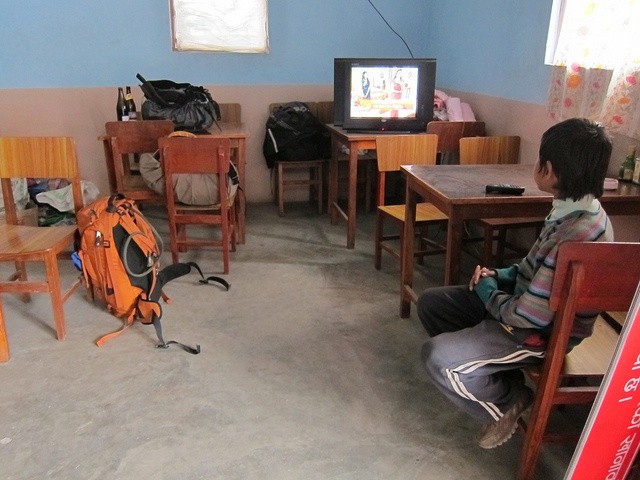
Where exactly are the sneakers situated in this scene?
[37,205,62,223]
[478,385,534,449]
[497,370,525,385]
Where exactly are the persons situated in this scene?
[417,117,614,450]
[390,69,404,118]
[360,71,372,100]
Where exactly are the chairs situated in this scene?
[512,241,640,480]
[373,134,449,269]
[217,103,241,165]
[426,121,485,232]
[158,137,237,274]
[0,136,96,339]
[318,101,372,212]
[269,101,324,217]
[460,136,547,268]
[105,121,188,253]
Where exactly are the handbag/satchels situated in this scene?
[142,81,222,133]
[138,131,247,225]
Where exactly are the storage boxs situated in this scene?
[0,199,40,227]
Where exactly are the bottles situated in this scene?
[116,88,129,126]
[617,147,635,183]
[125,88,137,122]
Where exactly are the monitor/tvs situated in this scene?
[334,58,436,134]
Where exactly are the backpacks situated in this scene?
[262,102,330,195]
[70,192,230,355]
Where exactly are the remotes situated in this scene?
[486,185,525,195]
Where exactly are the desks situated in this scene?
[400,164,640,319]
[97,122,250,245]
[324,123,427,250]
